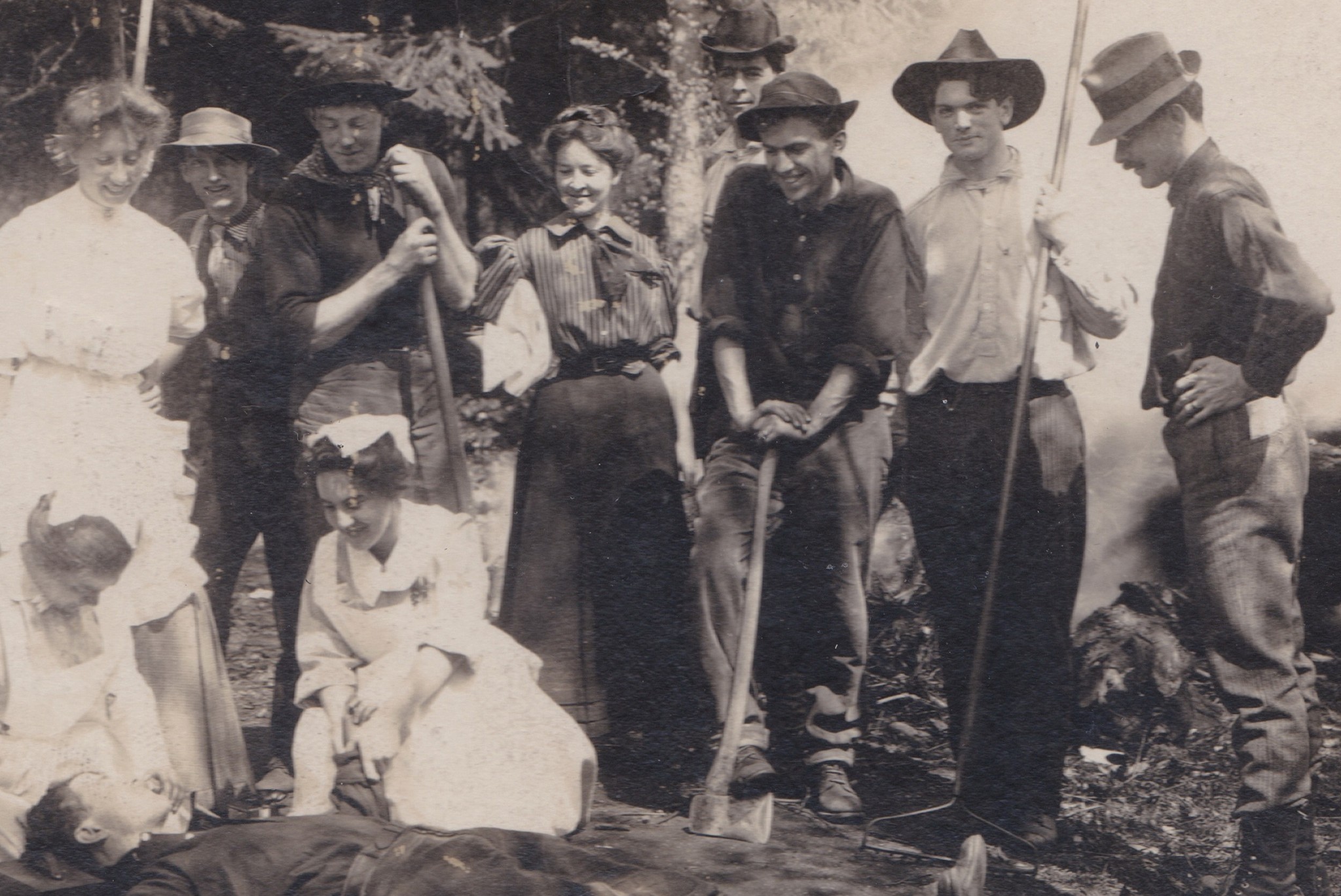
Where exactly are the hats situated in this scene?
[698,1,797,57]
[893,29,1046,131]
[276,46,419,108]
[157,107,280,160]
[736,72,860,142]
[1079,31,1202,147]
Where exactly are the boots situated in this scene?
[1225,813,1306,896]
[1297,806,1332,896]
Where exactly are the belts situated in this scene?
[342,819,410,896]
[560,352,650,378]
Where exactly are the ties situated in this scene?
[208,224,226,290]
[579,222,664,307]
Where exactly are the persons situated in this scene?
[475,103,718,738]
[900,28,1138,853]
[687,1,787,465]
[164,106,326,777]
[1104,32,1333,894]
[24,771,988,895]
[701,71,907,820]
[290,412,599,836]
[1,76,257,831]
[260,62,478,558]
[0,487,178,866]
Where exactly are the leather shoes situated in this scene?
[733,746,777,784]
[1015,814,1058,849]
[812,761,867,817]
